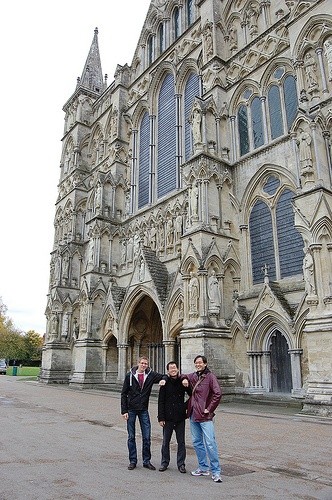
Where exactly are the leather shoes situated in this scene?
[128,463,136,470]
[178,464,186,473]
[143,463,156,470]
[159,462,169,471]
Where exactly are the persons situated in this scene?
[158,361,192,472]
[62,257,69,275]
[189,272,199,312]
[180,355,222,482]
[96,184,101,206]
[51,312,69,333]
[295,128,313,168]
[324,40,332,78]
[190,183,198,216]
[303,248,316,296]
[205,29,212,55]
[193,111,201,143]
[207,271,221,309]
[302,53,317,88]
[143,212,182,249]
[121,358,167,470]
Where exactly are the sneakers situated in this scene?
[191,468,210,476]
[211,474,223,482]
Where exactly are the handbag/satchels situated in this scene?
[184,398,189,409]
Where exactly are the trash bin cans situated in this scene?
[13,366,17,376]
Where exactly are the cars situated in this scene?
[0,360,7,375]
